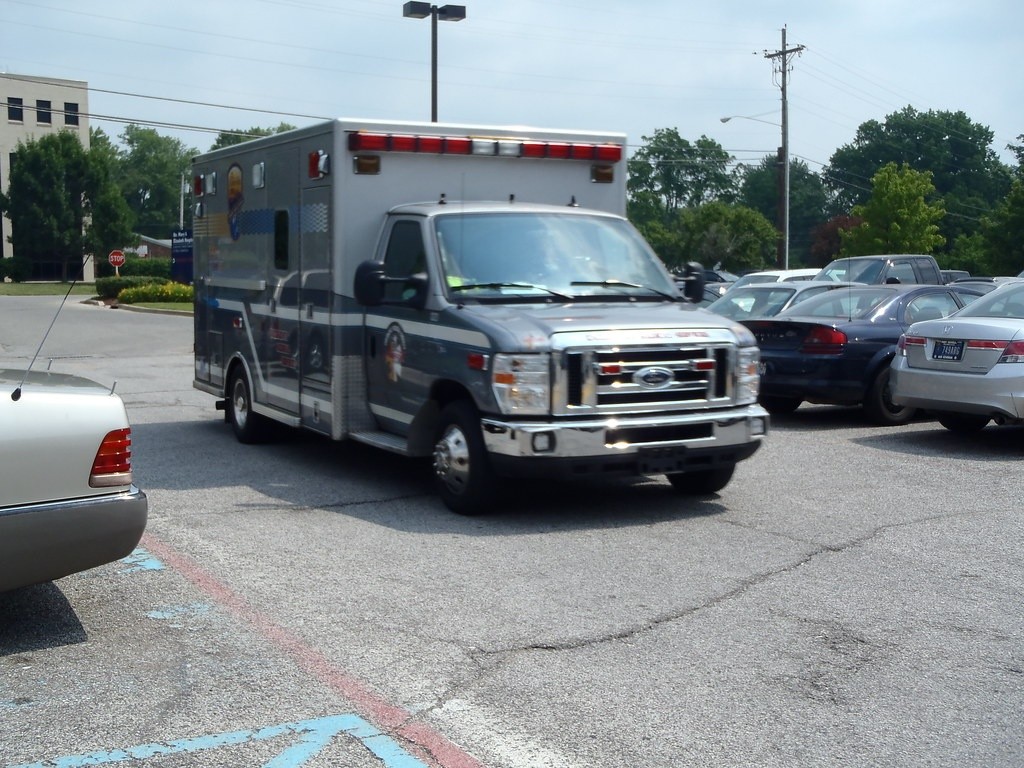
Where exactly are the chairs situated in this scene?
[913,306,943,322]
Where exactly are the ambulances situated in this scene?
[190,117,769,514]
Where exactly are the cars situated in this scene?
[671,254,1024,435]
[0,368,147,593]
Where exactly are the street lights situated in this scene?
[403,1,466,121]
[722,115,789,268]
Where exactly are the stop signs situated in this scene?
[109,250,125,268]
